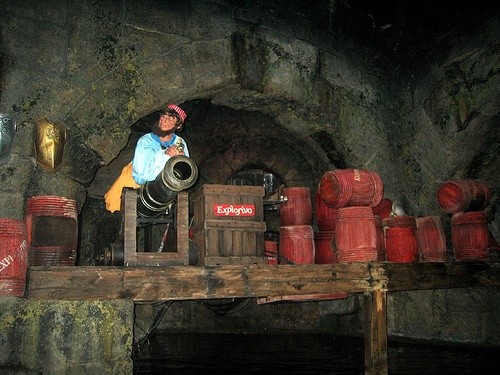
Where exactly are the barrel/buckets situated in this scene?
[318,168,383,208]
[314,230,336,264]
[451,211,491,261]
[279,187,312,226]
[24,195,78,266]
[279,223,315,266]
[373,198,392,219]
[414,216,447,262]
[437,180,491,214]
[383,217,418,263]
[263,230,279,265]
[0,219,29,297]
[373,215,385,261]
[335,206,378,263]
[315,194,337,232]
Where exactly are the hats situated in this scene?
[168,104,187,123]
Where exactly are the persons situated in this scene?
[131,104,191,253]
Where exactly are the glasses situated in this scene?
[161,114,174,122]
